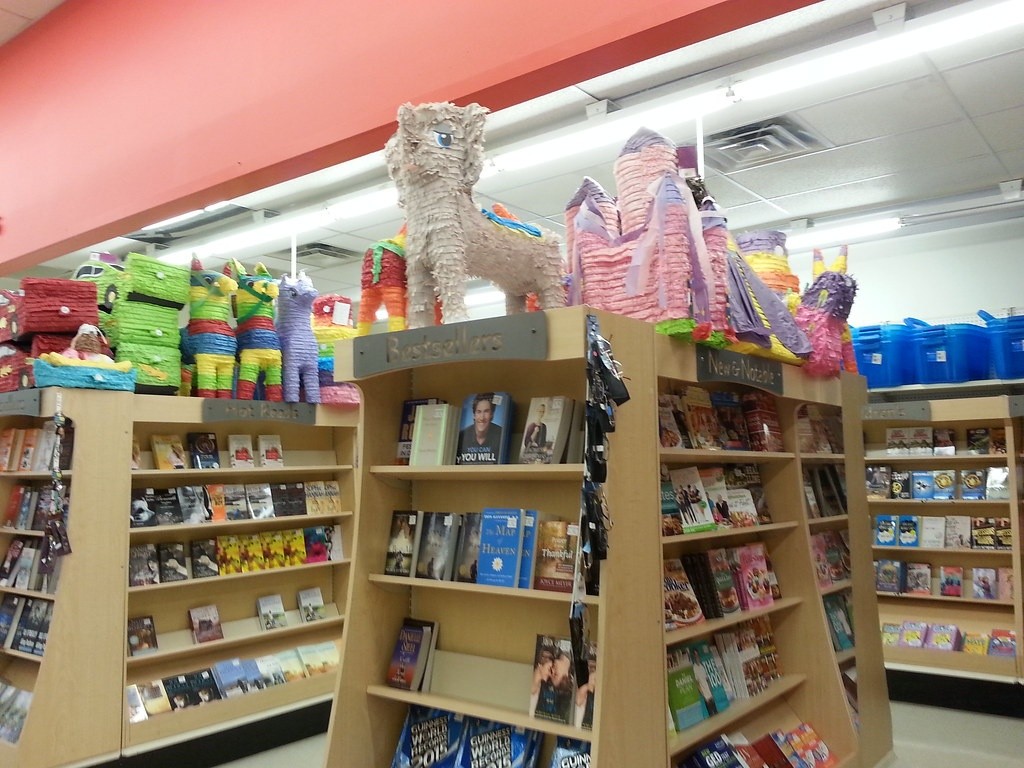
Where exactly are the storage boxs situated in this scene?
[840,309,1023,391]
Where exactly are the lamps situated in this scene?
[784,217,908,251]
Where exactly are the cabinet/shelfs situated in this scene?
[652,331,895,768]
[2,391,133,767]
[121,394,363,759]
[857,396,1023,683]
[318,303,666,767]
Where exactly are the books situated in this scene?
[863,419,1017,658]
[382,391,600,768]
[0,422,76,745]
[658,375,862,768]
[128,432,345,724]
